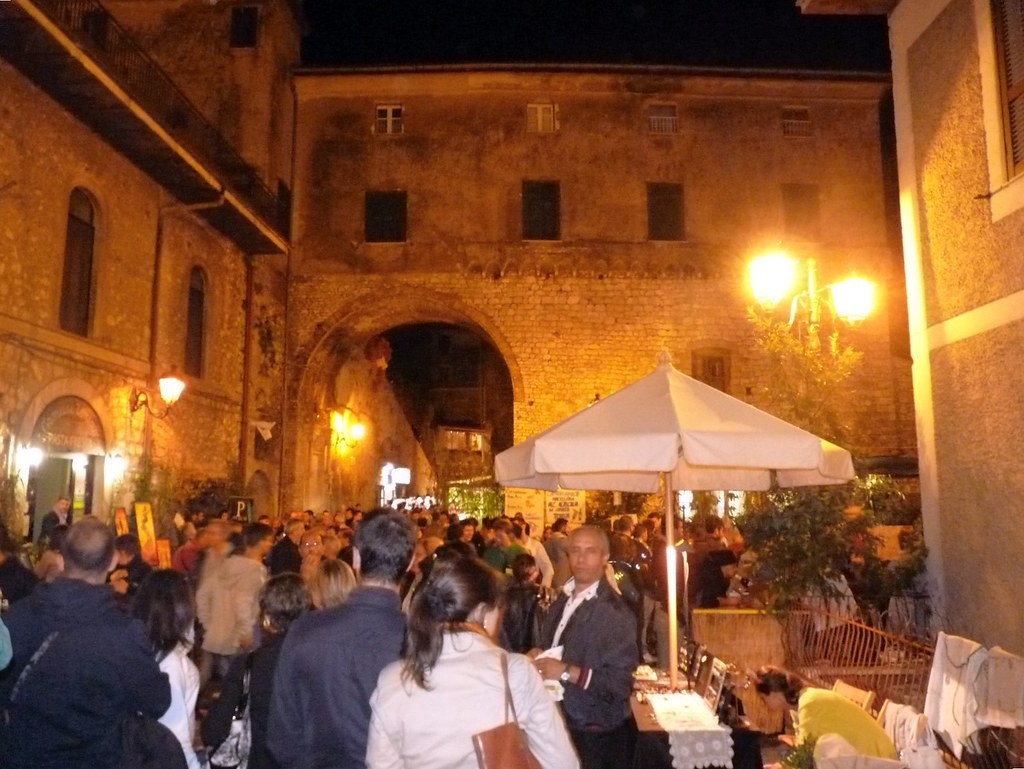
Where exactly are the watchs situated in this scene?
[560,663,572,686]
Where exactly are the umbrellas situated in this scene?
[494,351,857,691]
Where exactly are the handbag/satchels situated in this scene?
[532,585,555,646]
[207,649,252,769]
[472,654,543,769]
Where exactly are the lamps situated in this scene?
[130,364,187,420]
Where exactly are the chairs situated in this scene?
[703,656,727,711]
[876,699,924,748]
[777,679,876,751]
[686,642,706,686]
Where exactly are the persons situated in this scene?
[1,494,747,769]
[756,664,900,769]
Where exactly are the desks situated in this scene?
[632,667,687,693]
[630,690,735,769]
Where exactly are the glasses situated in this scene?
[280,532,287,538]
[301,541,322,548]
